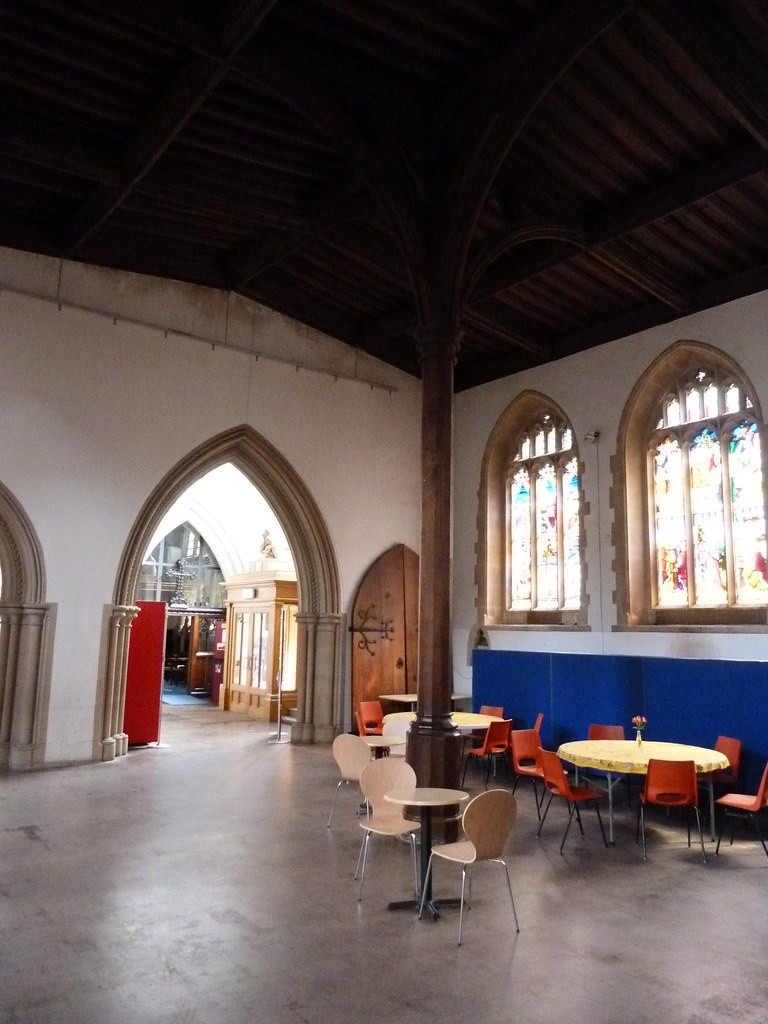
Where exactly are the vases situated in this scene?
[636,731,642,749]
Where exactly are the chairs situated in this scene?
[355,701,389,757]
[536,747,608,854]
[327,734,371,827]
[354,758,422,911]
[713,737,742,784]
[636,759,707,864]
[511,729,568,821]
[714,763,768,857]
[586,724,631,811]
[418,789,520,946]
[460,706,503,769]
[462,719,512,791]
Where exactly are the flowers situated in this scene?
[632,716,648,746]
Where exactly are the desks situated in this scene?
[557,741,730,846]
[360,736,406,760]
[378,694,472,712]
[384,788,469,922]
[382,712,504,781]
